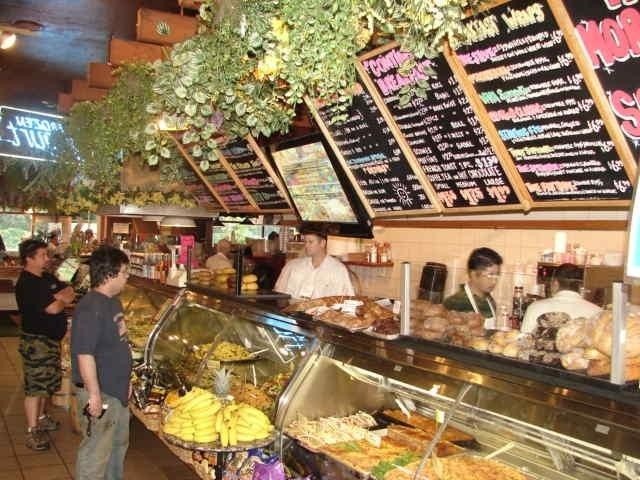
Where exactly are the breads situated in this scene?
[292,297,640,389]
[190,267,259,291]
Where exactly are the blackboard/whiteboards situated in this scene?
[156,0,639,217]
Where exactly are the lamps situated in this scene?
[143,216,197,228]
[0,27,16,50]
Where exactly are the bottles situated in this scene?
[363,245,392,265]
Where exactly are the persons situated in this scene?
[273,232,356,305]
[205,239,235,270]
[442,246,503,330]
[85,229,97,247]
[520,262,604,335]
[47,235,59,248]
[69,245,136,480]
[14,238,75,451]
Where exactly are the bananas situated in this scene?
[152,386,273,449]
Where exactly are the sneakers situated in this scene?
[24,415,60,450]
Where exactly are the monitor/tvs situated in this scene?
[264,132,373,239]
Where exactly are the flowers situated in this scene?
[144,0,488,171]
[52,188,197,214]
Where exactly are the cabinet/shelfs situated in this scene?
[538,263,624,295]
